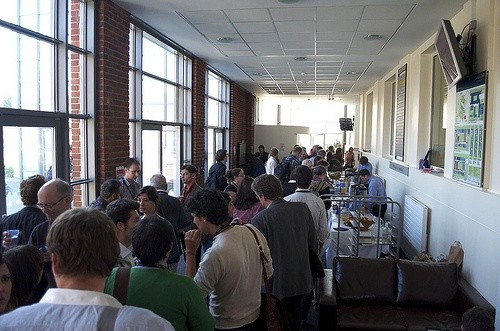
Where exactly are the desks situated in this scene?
[326,196,381,269]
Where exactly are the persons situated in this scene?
[347,158,374,175]
[249,145,374,177]
[178,189,276,331]
[0,254,14,314]
[0,245,44,315]
[264,146,281,175]
[359,168,388,218]
[0,177,74,294]
[283,166,327,257]
[89,180,122,208]
[0,174,47,249]
[135,184,175,272]
[250,152,266,181]
[216,191,235,223]
[118,156,146,201]
[345,146,358,173]
[232,177,264,226]
[176,162,204,274]
[104,196,143,265]
[206,148,229,191]
[151,173,188,272]
[251,174,319,331]
[224,167,248,201]
[0,209,176,331]
[309,166,331,209]
[282,144,303,177]
[108,213,210,331]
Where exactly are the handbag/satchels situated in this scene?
[267,309,283,331]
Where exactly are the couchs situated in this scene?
[318,256,496,331]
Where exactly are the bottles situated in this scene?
[382,223,392,244]
[360,198,366,216]
[333,179,345,194]
[371,217,382,241]
[332,198,338,215]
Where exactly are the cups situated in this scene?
[7,230,19,248]
[341,187,346,193]
[342,208,350,222]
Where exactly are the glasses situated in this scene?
[36,196,68,208]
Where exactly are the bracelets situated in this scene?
[183,249,197,255]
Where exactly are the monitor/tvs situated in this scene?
[435,19,467,89]
